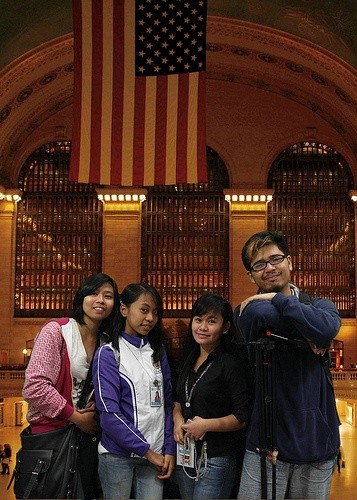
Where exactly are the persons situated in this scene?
[172,293,252,500]
[22,273,120,500]
[0,444,12,475]
[92,282,175,500]
[336,447,343,474]
[233,231,341,500]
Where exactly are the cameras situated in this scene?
[184,419,206,440]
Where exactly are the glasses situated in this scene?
[240,254,288,272]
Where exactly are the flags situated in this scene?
[68,0,208,184]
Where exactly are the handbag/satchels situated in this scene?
[8,424,85,500]
